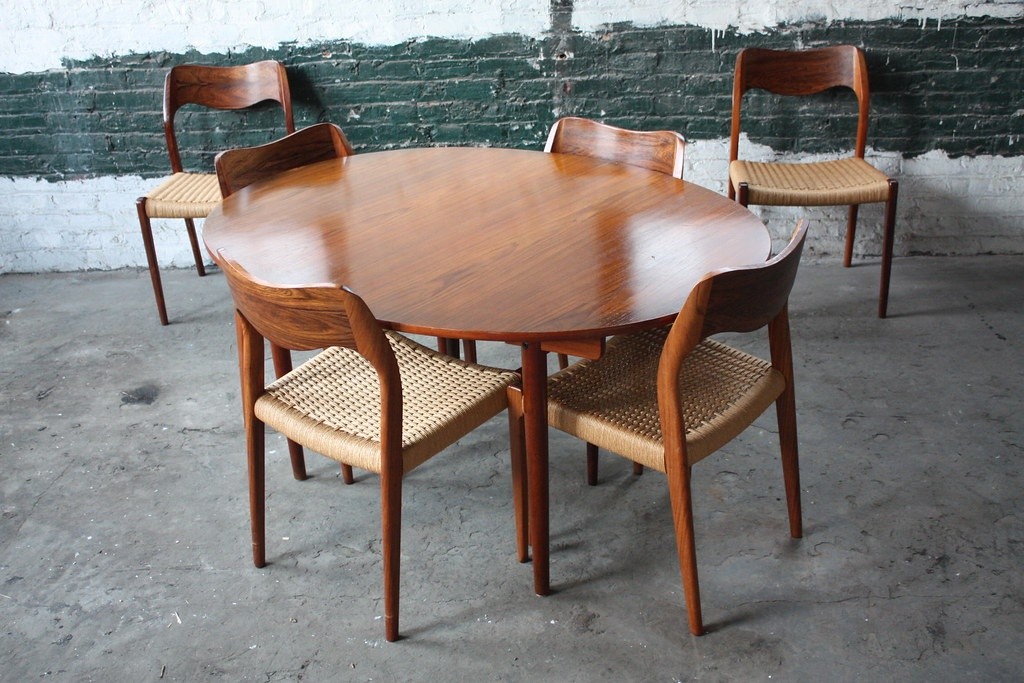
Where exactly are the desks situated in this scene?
[204,145,771,599]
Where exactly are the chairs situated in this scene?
[505,224,808,635]
[216,248,526,642]
[213,120,445,483]
[135,59,298,325]
[462,116,687,487]
[729,46,898,318]
